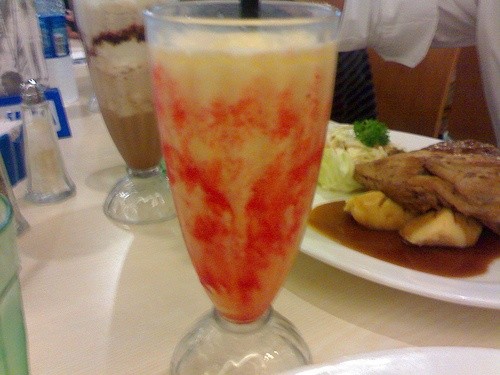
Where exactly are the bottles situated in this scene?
[18,81,75,204]
[34,0,79,109]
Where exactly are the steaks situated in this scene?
[353,139,500,236]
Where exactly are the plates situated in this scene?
[293,346,500,375]
[299,124,500,314]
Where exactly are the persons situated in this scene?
[268,0,499,144]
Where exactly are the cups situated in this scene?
[0,192,29,375]
[139,0,312,375]
[72,0,198,227]
